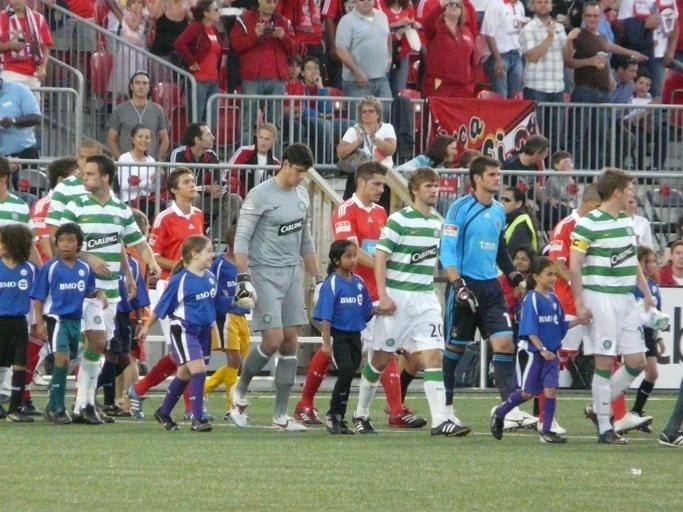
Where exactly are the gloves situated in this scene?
[508,270,528,302]
[312,274,324,305]
[232,272,258,311]
[450,276,480,314]
[638,297,669,331]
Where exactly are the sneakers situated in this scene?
[1,382,683,449]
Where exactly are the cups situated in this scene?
[595,51,611,71]
[263,20,274,34]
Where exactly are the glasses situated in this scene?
[501,197,515,203]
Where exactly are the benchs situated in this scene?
[138,332,325,373]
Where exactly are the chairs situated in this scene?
[660,0,683,132]
[524,184,682,247]
[51,1,572,154]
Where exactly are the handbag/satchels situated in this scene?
[337,126,376,174]
